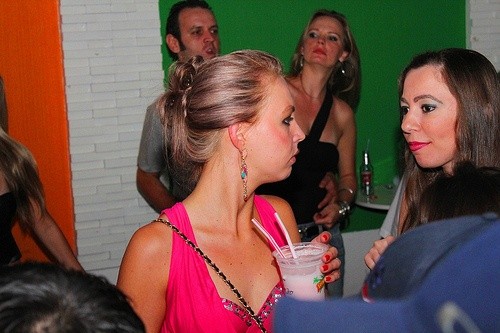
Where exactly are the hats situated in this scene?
[274,207,500,333]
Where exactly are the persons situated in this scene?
[0,0,500,333]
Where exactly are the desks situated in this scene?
[353,183,401,210]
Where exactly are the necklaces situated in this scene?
[300,73,320,98]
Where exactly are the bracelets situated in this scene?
[338,187,355,198]
[336,200,350,217]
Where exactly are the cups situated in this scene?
[273,243,327,302]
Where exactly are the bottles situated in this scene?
[360,152,374,199]
